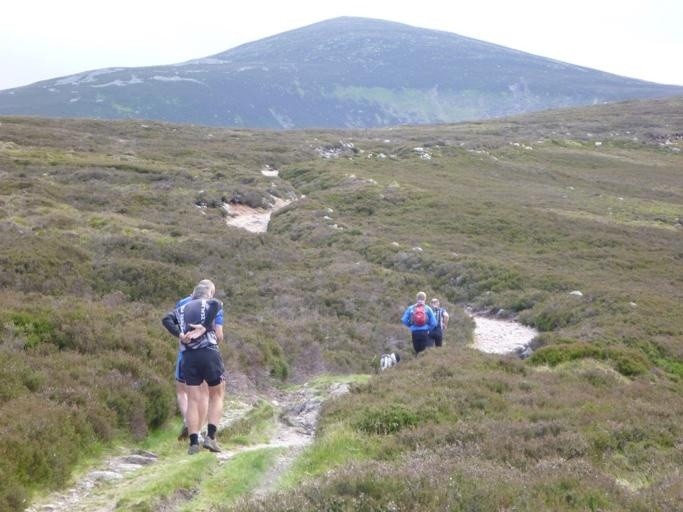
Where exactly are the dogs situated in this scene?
[379,352,400,371]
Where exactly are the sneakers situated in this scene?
[203,437,220,452]
[178,428,188,441]
[188,445,199,454]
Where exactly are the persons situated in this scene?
[174,278,216,442]
[400,291,436,355]
[428,298,449,347]
[161,284,227,454]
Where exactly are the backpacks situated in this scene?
[411,301,428,325]
